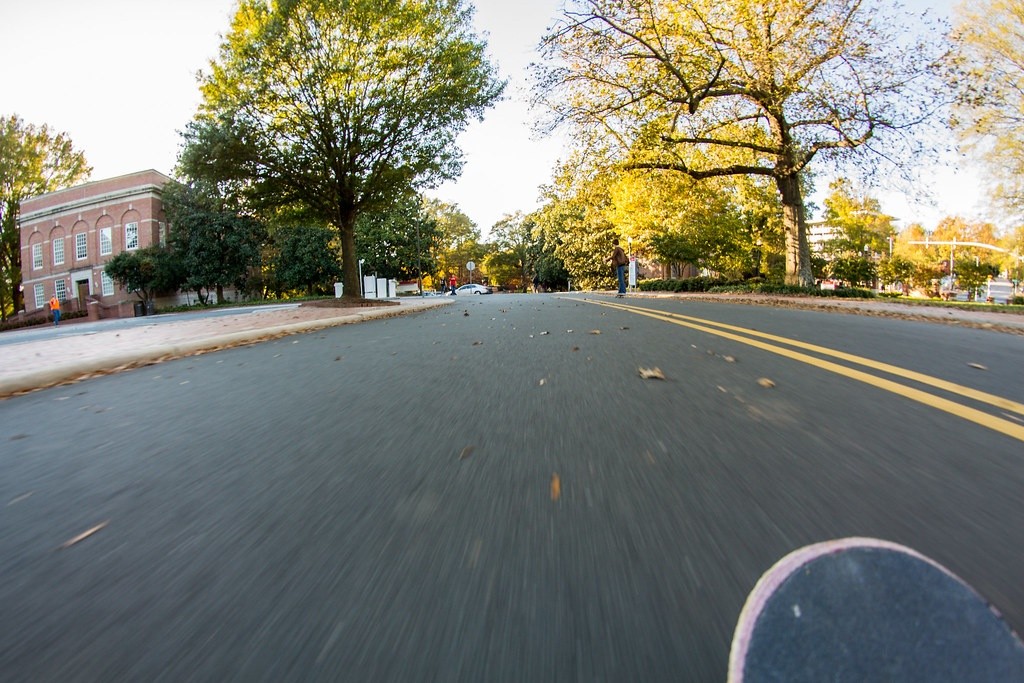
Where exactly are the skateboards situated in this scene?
[615,293,626,298]
[725,536,1024,683]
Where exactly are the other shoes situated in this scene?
[617,293,626,295]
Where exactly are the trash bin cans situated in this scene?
[134,301,146,317]
[145,302,154,316]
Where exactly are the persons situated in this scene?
[50,294,61,325]
[533,274,540,293]
[450,274,457,295]
[605,239,628,294]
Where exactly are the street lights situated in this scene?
[757,240,763,276]
[627,236,632,262]
[359,259,365,297]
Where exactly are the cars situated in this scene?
[446,283,493,294]
